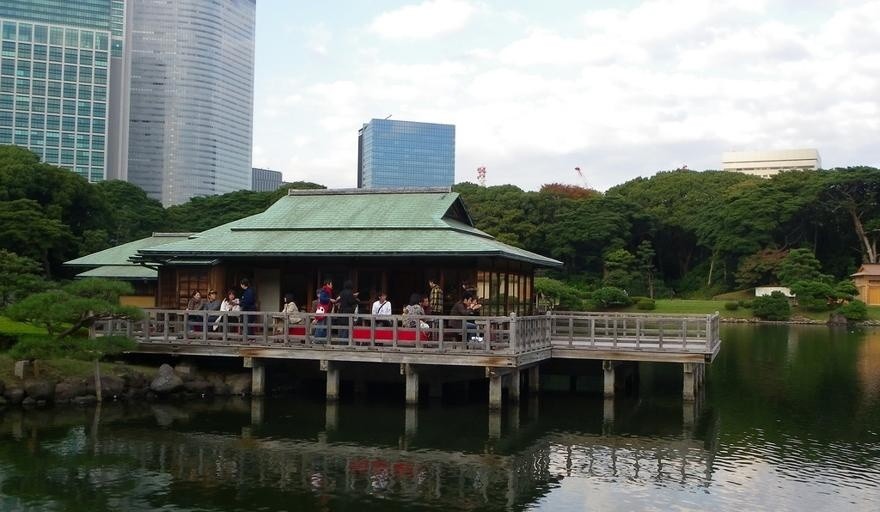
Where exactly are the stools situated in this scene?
[288,326,306,343]
[443,326,461,351]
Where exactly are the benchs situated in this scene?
[351,325,428,350]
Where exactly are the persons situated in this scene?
[214,457,427,497]
[185,277,484,347]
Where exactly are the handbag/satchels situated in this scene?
[212,314,225,331]
[315,303,331,321]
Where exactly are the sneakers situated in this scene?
[248,339,256,343]
[312,339,327,344]
